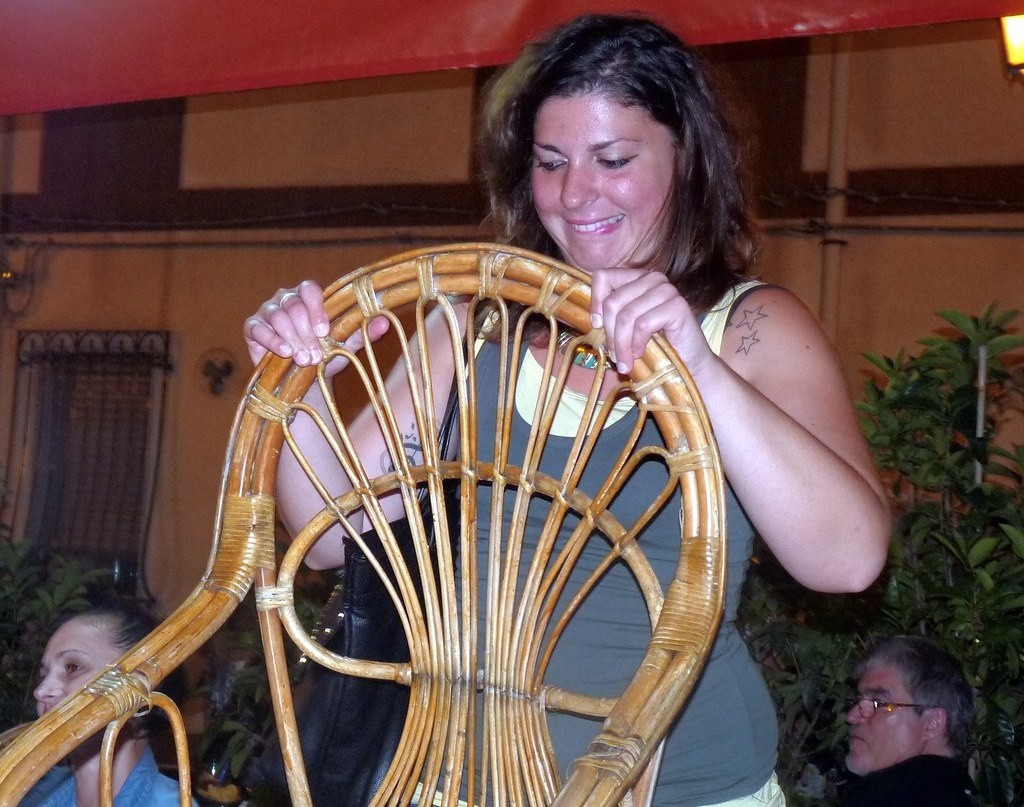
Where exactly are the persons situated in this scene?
[240,9,893,807]
[828,634,986,806]
[18,599,196,806]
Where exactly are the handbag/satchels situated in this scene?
[233,491,463,807]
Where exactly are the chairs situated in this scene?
[0,241,729,807]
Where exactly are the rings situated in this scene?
[262,302,279,317]
[244,317,261,339]
[277,288,299,312]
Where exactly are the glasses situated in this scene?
[844,698,923,719]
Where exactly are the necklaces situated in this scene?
[554,326,617,370]
